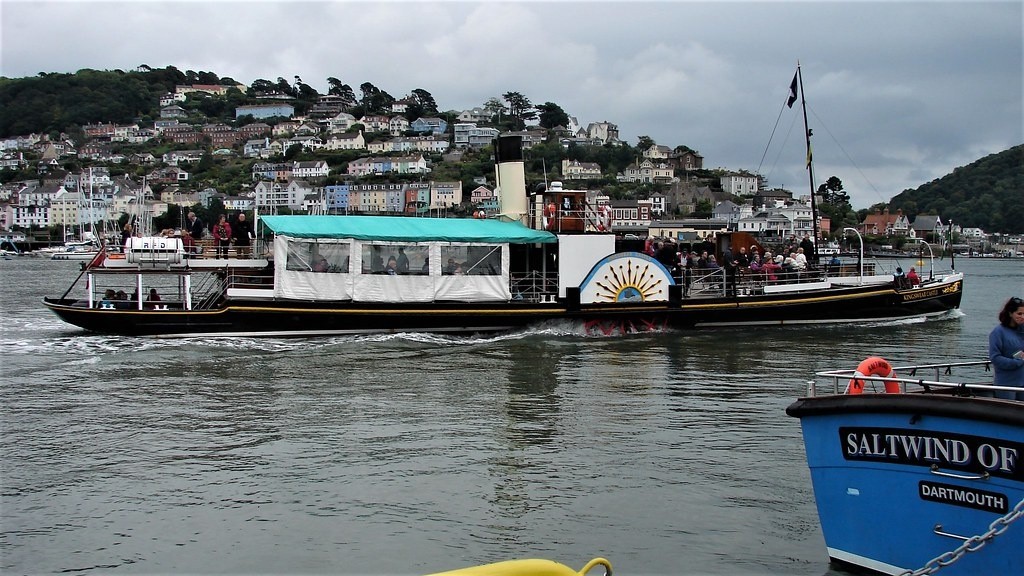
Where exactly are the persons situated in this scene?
[907,267,920,285]
[213,214,231,259]
[643,234,814,297]
[120,224,132,252]
[828,252,840,277]
[422,257,429,275]
[187,212,203,239]
[561,199,569,216]
[99,288,163,310]
[160,228,196,259]
[448,258,480,275]
[231,213,256,259]
[372,246,409,275]
[310,254,328,272]
[989,297,1024,401]
[894,267,905,289]
[341,255,349,273]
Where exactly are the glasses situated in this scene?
[1013,299,1024,304]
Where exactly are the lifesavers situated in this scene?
[596,205,612,231]
[849,358,900,394]
[542,202,556,231]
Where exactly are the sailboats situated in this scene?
[1,165,268,279]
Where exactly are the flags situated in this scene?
[805,139,813,169]
[787,72,797,109]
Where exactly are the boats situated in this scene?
[39,54,966,338]
[785,359,1024,576]
